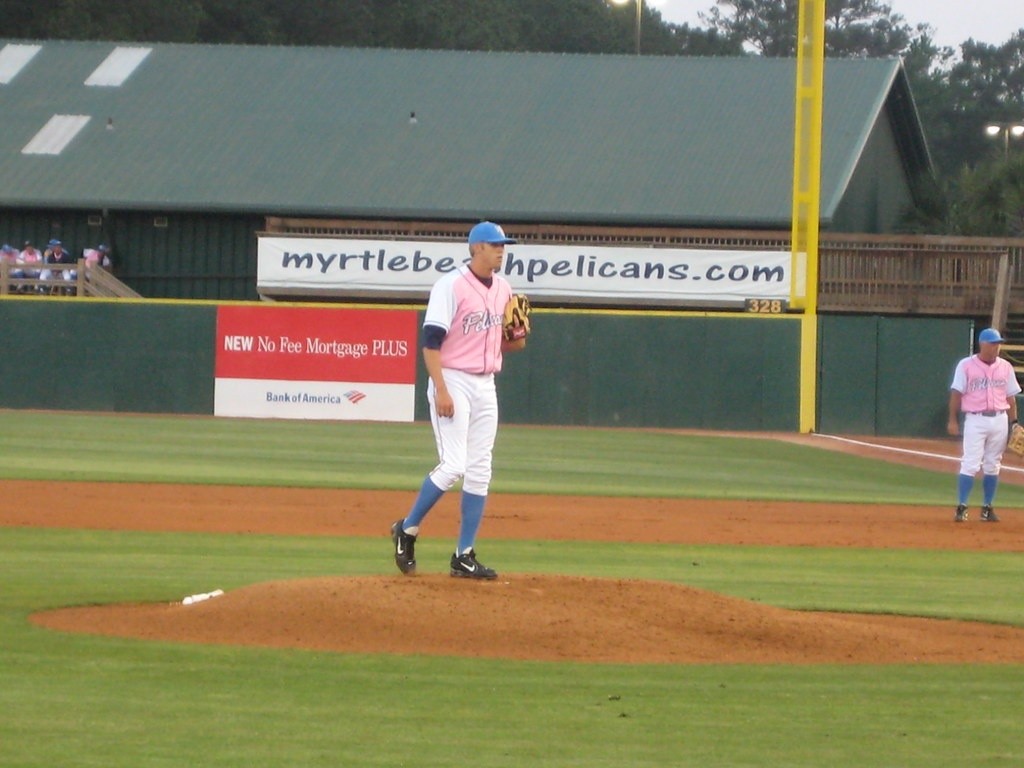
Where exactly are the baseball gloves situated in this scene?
[1007,423,1024,456]
[503,294,531,340]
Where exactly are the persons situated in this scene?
[0,240,110,295]
[947,329,1024,522]
[390,223,531,579]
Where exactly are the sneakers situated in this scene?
[450,548,498,580]
[979,505,1000,522]
[954,504,969,522]
[391,518,419,574]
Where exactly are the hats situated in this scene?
[2,244,12,254]
[54,241,62,247]
[47,239,57,248]
[468,221,517,244]
[99,245,107,252]
[25,241,32,247]
[979,328,1005,343]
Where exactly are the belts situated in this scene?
[972,411,1004,417]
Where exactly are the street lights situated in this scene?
[984,120,1024,162]
